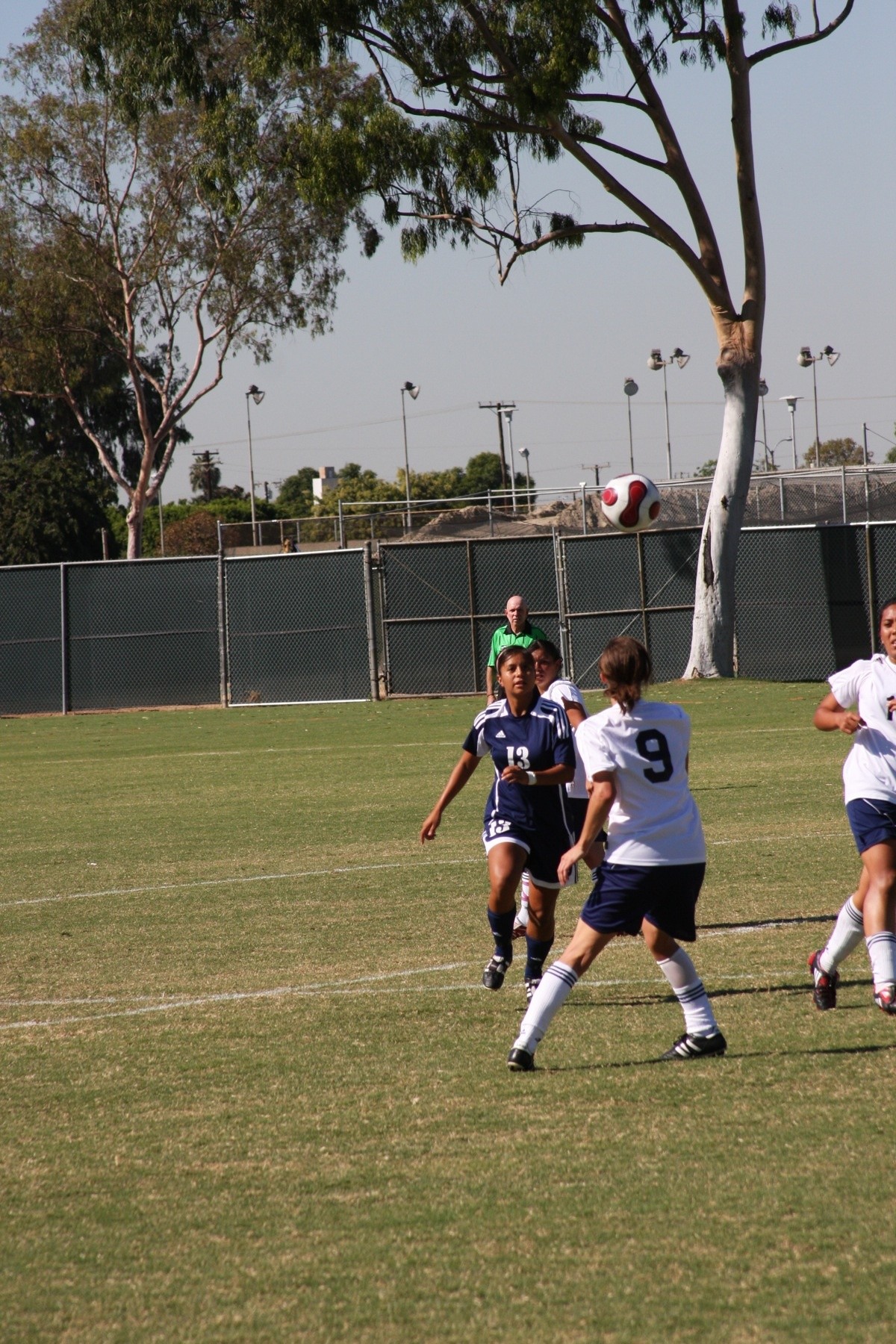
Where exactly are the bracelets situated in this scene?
[526,771,536,785]
[486,693,493,697]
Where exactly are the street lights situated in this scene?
[755,437,793,472]
[758,377,769,472]
[797,345,841,466]
[245,384,265,547]
[579,482,588,535]
[401,381,421,532]
[518,447,532,514]
[497,406,519,515]
[623,377,639,474]
[779,395,804,469]
[647,347,691,478]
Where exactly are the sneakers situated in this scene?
[873,984,896,1016]
[660,1025,727,1060]
[524,976,543,1003]
[512,916,527,940]
[483,954,513,991]
[507,1047,536,1072]
[806,950,840,1012]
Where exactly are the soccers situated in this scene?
[599,473,661,534]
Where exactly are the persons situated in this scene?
[510,644,606,942]
[808,597,896,1012]
[420,646,576,1007]
[507,638,728,1072]
[486,596,547,706]
[279,537,300,554]
